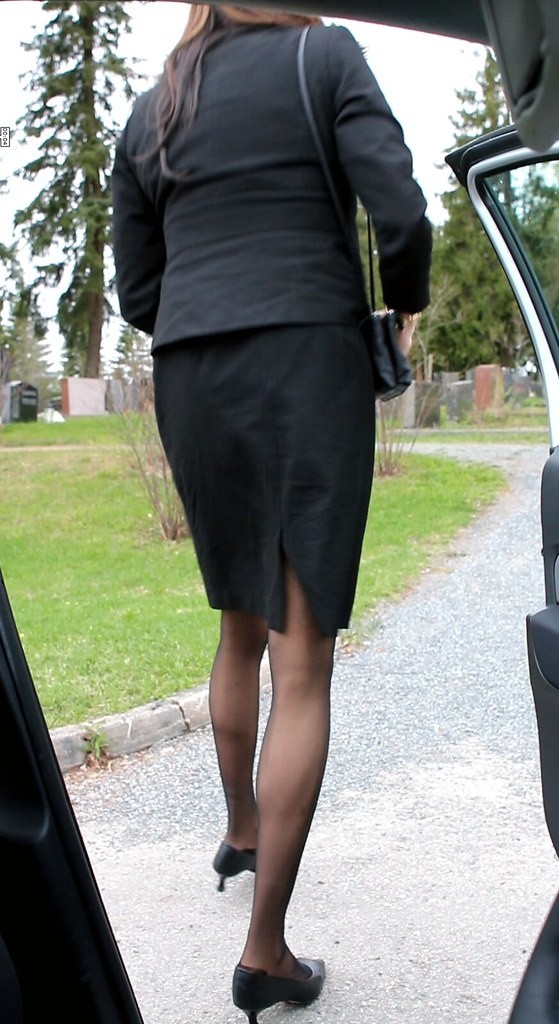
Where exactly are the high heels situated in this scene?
[211,842,256,891]
[231,955,327,1024]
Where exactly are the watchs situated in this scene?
[393,312,424,330]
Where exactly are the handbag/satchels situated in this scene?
[370,307,411,396]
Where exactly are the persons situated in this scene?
[114,3,434,1024]
